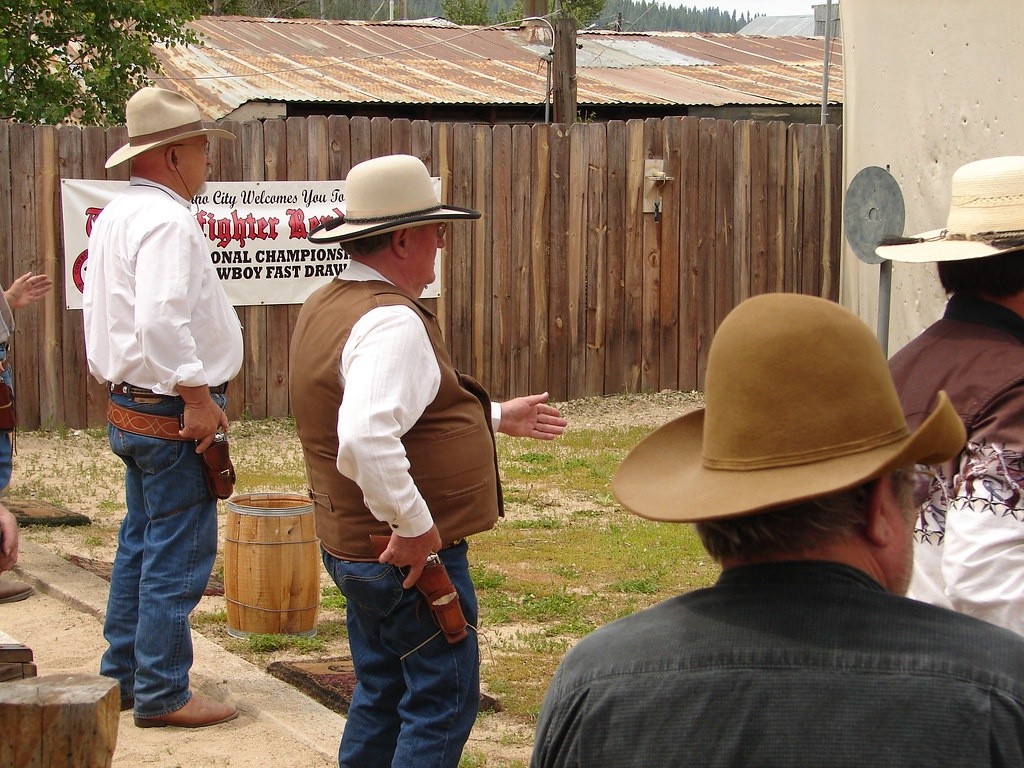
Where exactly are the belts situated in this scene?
[109,382,225,398]
[106,400,195,441]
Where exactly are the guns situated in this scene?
[179,412,226,447]
[369,534,443,579]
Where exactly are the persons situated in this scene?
[523,292,1024,768]
[83,88,243,726]
[888,157,1024,638]
[0,271,53,604]
[289,154,567,768]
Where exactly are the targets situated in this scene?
[843,165,905,265]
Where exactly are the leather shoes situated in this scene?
[135,694,238,728]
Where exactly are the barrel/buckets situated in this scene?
[223,492,321,640]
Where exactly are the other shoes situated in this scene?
[0,579,32,603]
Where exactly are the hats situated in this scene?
[876,156,1024,263]
[105,87,237,168]
[308,154,482,243]
[611,292,968,522]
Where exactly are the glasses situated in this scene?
[888,467,934,506]
[165,142,210,156]
[411,223,446,238]
